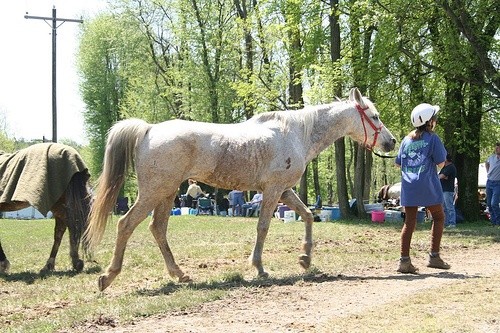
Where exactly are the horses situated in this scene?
[0,143,93,275]
[78,87,395,292]
[373,181,401,201]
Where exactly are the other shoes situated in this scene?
[427,254,451,270]
[397,257,419,275]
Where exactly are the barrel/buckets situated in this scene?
[332,207,340,221]
[371,211,384,223]
[279,206,290,218]
[417,211,425,222]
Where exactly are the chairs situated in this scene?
[275,204,290,220]
[246,202,261,217]
[196,198,213,216]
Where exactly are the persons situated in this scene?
[485,143,500,225]
[186,179,202,207]
[240,191,262,217]
[395,102,451,274]
[439,154,456,228]
[173,185,181,208]
[228,190,242,216]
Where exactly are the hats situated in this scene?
[410,103,440,127]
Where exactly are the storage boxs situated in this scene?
[322,207,341,220]
